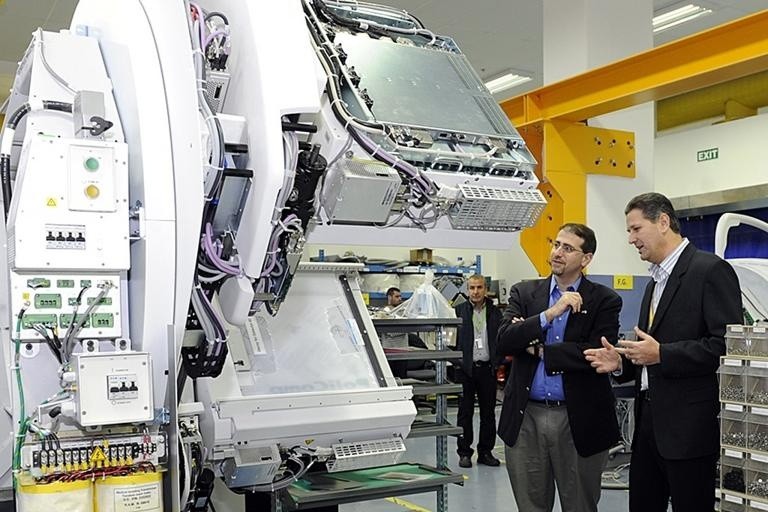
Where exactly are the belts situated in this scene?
[640,389,652,401]
[528,398,565,408]
[473,360,491,367]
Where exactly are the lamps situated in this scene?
[484,69,535,96]
[652,1,713,39]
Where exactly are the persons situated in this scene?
[446,275,500,467]
[385,287,402,306]
[583,192,746,512]
[496,222,624,512]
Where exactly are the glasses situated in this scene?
[553,242,585,253]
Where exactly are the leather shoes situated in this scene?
[459,456,472,468]
[477,454,500,466]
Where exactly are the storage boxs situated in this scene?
[714,324,768,511]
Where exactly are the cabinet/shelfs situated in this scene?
[245,248,483,511]
